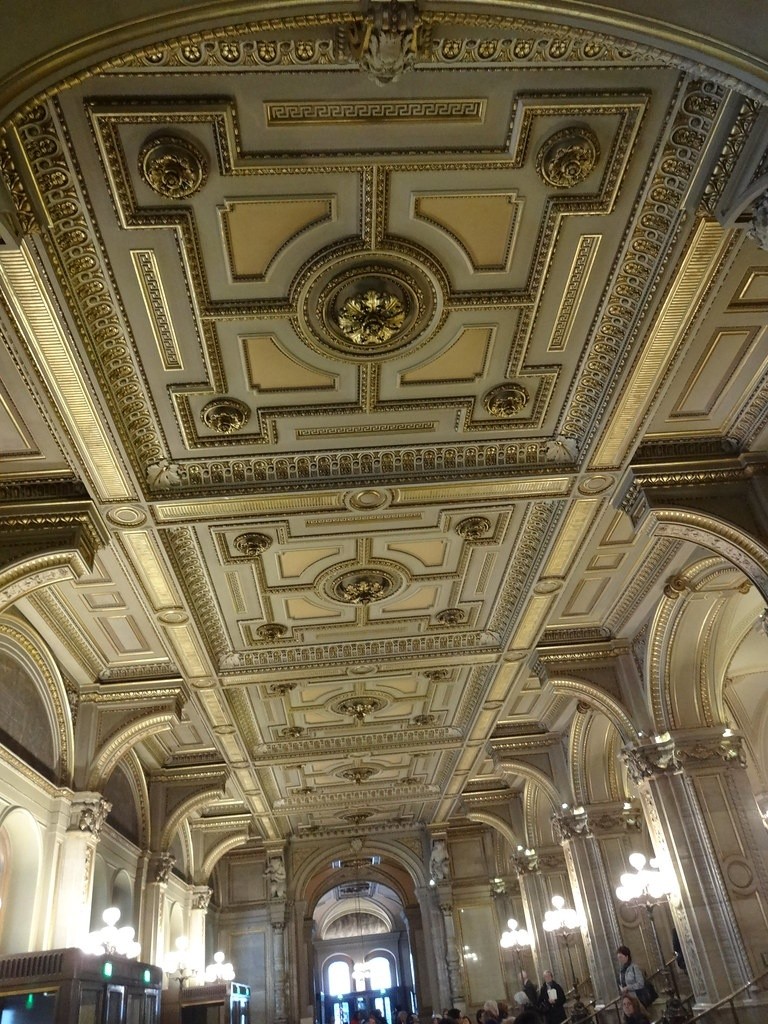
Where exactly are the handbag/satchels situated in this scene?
[633,964,659,1008]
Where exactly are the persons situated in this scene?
[617,946,651,1024]
[350,971,566,1024]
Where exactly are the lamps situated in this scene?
[617,851,693,1023]
[543,895,594,1024]
[352,962,374,981]
[80,907,142,1024]
[162,938,236,1024]
[500,919,530,985]
